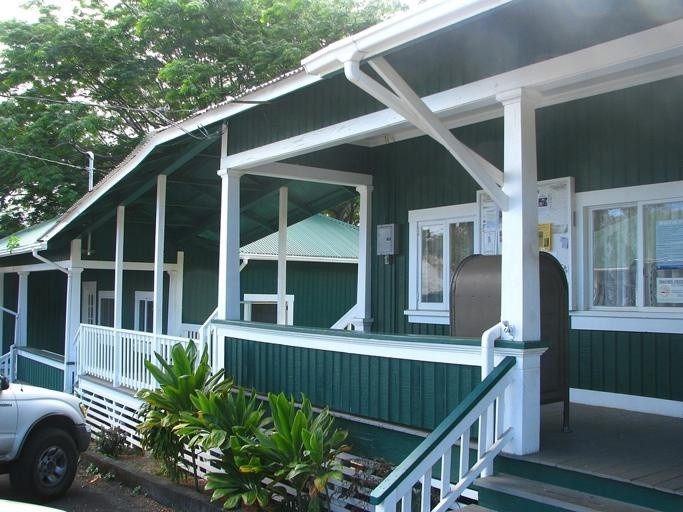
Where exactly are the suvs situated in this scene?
[0,368,92,502]
